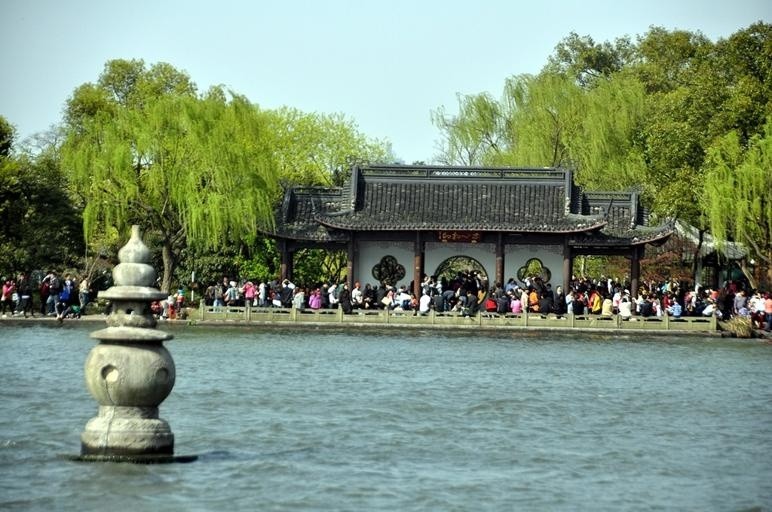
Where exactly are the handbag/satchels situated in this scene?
[224,293,240,302]
[381,297,390,306]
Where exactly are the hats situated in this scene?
[354,282,361,287]
[230,281,237,287]
[284,281,290,284]
[52,269,58,276]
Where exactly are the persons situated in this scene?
[167,270,772,332]
[0,269,93,321]
